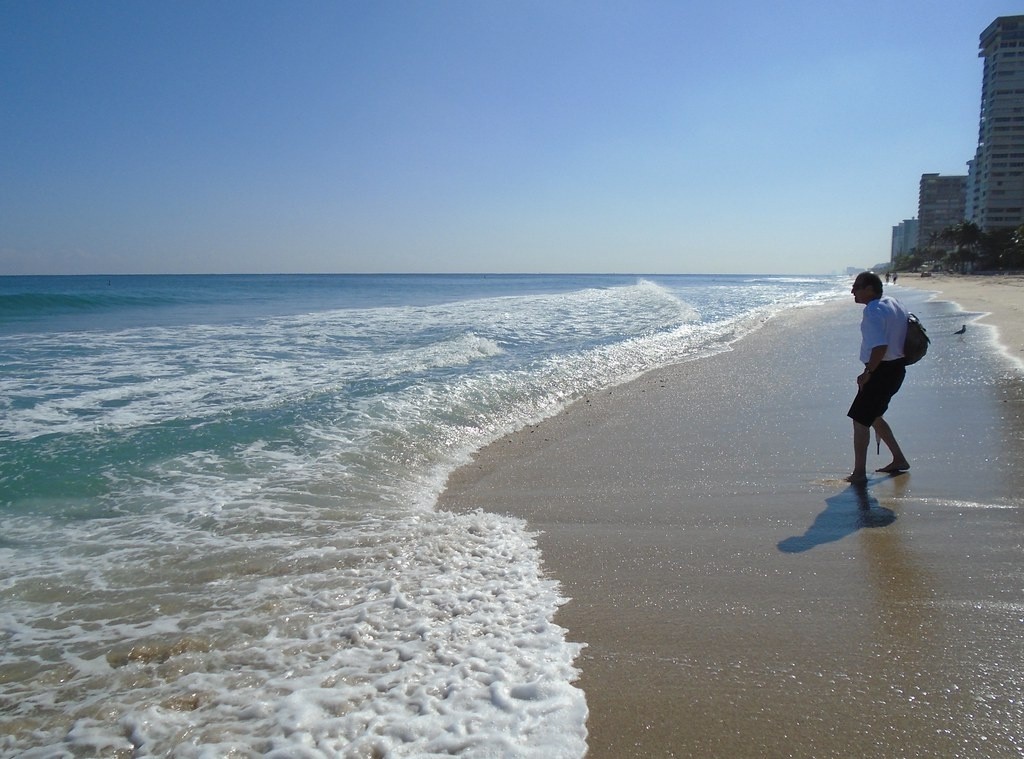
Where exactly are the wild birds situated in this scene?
[953,326,967,335]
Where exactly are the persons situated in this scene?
[840,271,910,484]
[885,271,890,285]
[892,272,898,286]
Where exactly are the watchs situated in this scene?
[864,368,872,376]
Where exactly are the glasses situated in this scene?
[853,285,865,290]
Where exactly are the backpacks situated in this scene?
[892,296,930,366]
